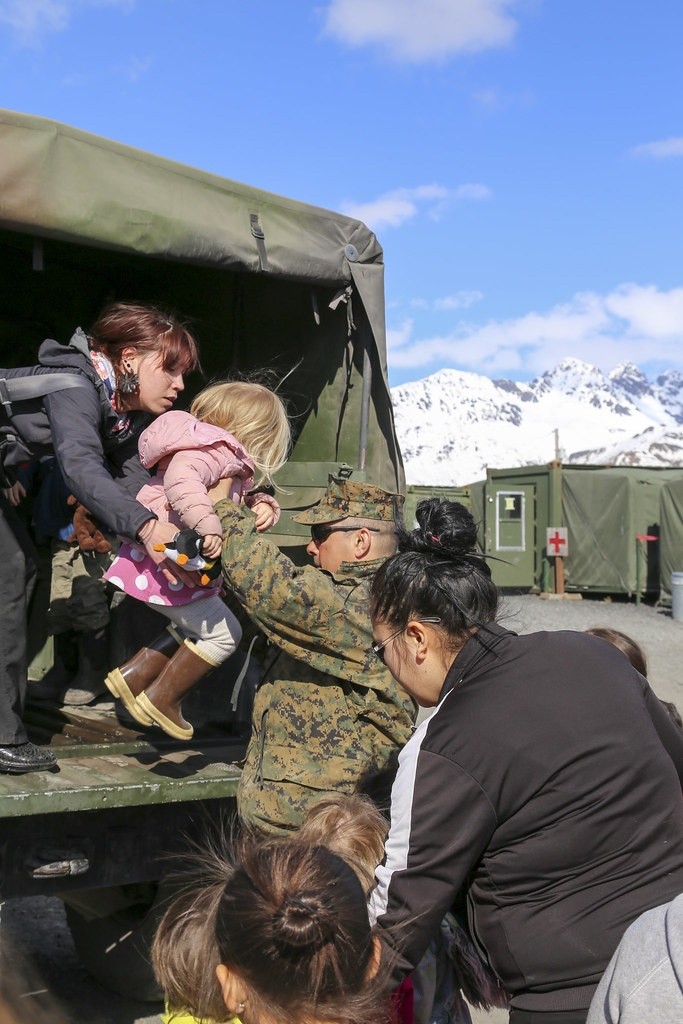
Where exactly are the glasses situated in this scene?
[311,526,380,544]
[371,617,441,660]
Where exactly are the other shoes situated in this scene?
[0,744,57,772]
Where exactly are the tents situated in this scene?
[403,462,683,610]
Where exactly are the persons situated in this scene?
[1,303,471,1024]
[363,497,682,1024]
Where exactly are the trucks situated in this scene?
[0,108,411,1004]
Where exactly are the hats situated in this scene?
[290,477,406,525]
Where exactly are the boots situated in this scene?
[136,637,222,740]
[59,625,111,705]
[27,629,78,699]
[102,624,185,727]
